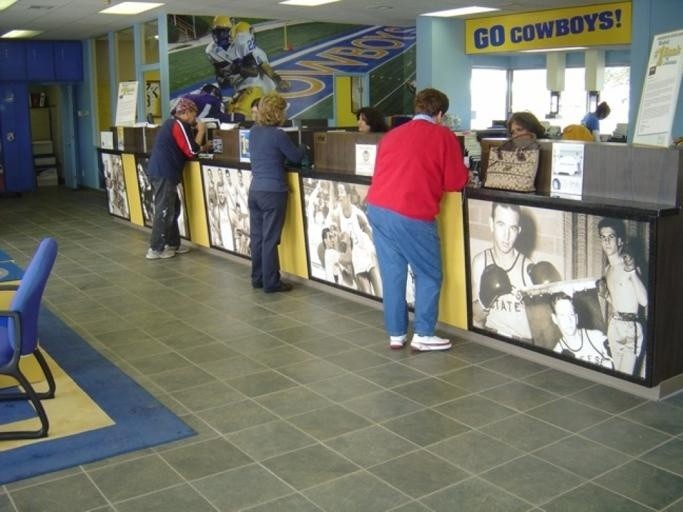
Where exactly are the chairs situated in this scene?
[0,237,58,440]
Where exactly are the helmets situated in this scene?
[201,83,224,108]
[227,22,255,44]
[210,16,235,45]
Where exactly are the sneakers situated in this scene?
[410,333,452,352]
[145,247,176,259]
[164,244,191,254]
[253,281,292,293]
[390,333,409,349]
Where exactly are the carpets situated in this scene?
[0,248,197,486]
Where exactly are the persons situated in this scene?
[305,182,382,298]
[357,107,388,132]
[203,168,250,256]
[363,88,469,352]
[248,93,311,293]
[179,16,291,128]
[508,112,549,140]
[473,200,649,377]
[145,98,214,261]
[583,102,610,142]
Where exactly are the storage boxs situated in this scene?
[32,141,59,187]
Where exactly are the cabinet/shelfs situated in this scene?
[0,40,83,83]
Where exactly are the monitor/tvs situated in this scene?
[299,118,330,167]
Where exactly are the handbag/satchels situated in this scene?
[484,146,541,192]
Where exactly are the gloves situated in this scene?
[620,243,637,272]
[478,263,512,310]
[528,261,562,285]
[596,276,609,299]
